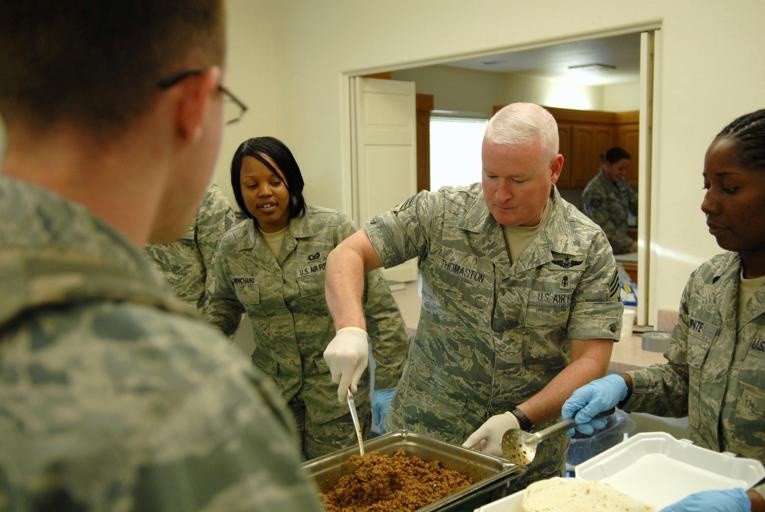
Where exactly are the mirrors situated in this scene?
[351,35,646,326]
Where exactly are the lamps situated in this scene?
[567,62,616,80]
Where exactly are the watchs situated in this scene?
[509,405,536,434]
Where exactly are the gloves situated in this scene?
[660,487,752,512]
[370,387,397,436]
[560,373,628,436]
[461,410,521,458]
[323,327,369,404]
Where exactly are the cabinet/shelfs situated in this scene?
[616,110,639,190]
[491,103,616,212]
[353,73,433,233]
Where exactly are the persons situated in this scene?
[0,0,325,512]
[581,147,637,255]
[323,101,624,459]
[196,136,409,464]
[561,108,765,512]
[143,181,238,317]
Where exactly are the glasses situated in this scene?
[155,67,251,128]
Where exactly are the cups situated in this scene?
[619,309,636,337]
